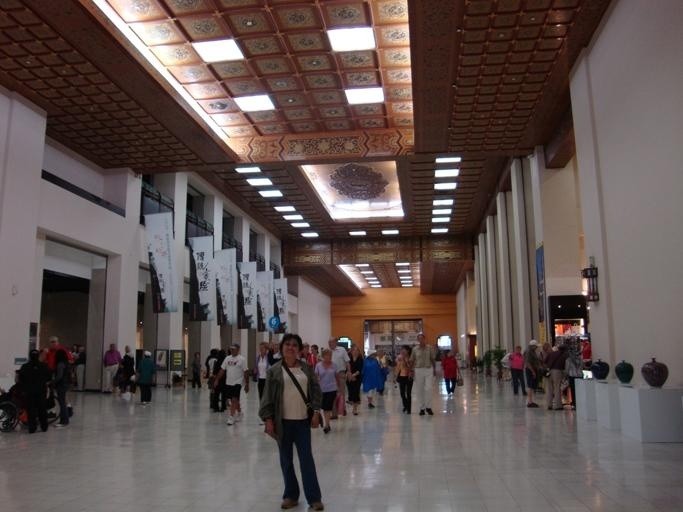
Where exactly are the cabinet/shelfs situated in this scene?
[574,379,596,421]
[617,384,683,443]
[595,382,620,430]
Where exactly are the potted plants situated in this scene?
[493,346,507,383]
[484,350,493,377]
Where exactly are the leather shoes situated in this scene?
[282,498,298,508]
[310,501,323,510]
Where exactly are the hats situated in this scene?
[530,340,542,347]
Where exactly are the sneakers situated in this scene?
[402,406,434,416]
[320,407,360,434]
[527,403,537,407]
[226,410,244,425]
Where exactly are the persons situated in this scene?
[253,333,387,433]
[19,348,54,434]
[441,349,460,396]
[122,344,137,399]
[102,343,122,395]
[258,333,327,511]
[70,343,85,393]
[191,351,203,390]
[393,334,437,416]
[38,336,76,418]
[205,343,250,425]
[509,335,577,410]
[49,348,72,428]
[136,350,157,406]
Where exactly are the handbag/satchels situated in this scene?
[457,378,464,385]
